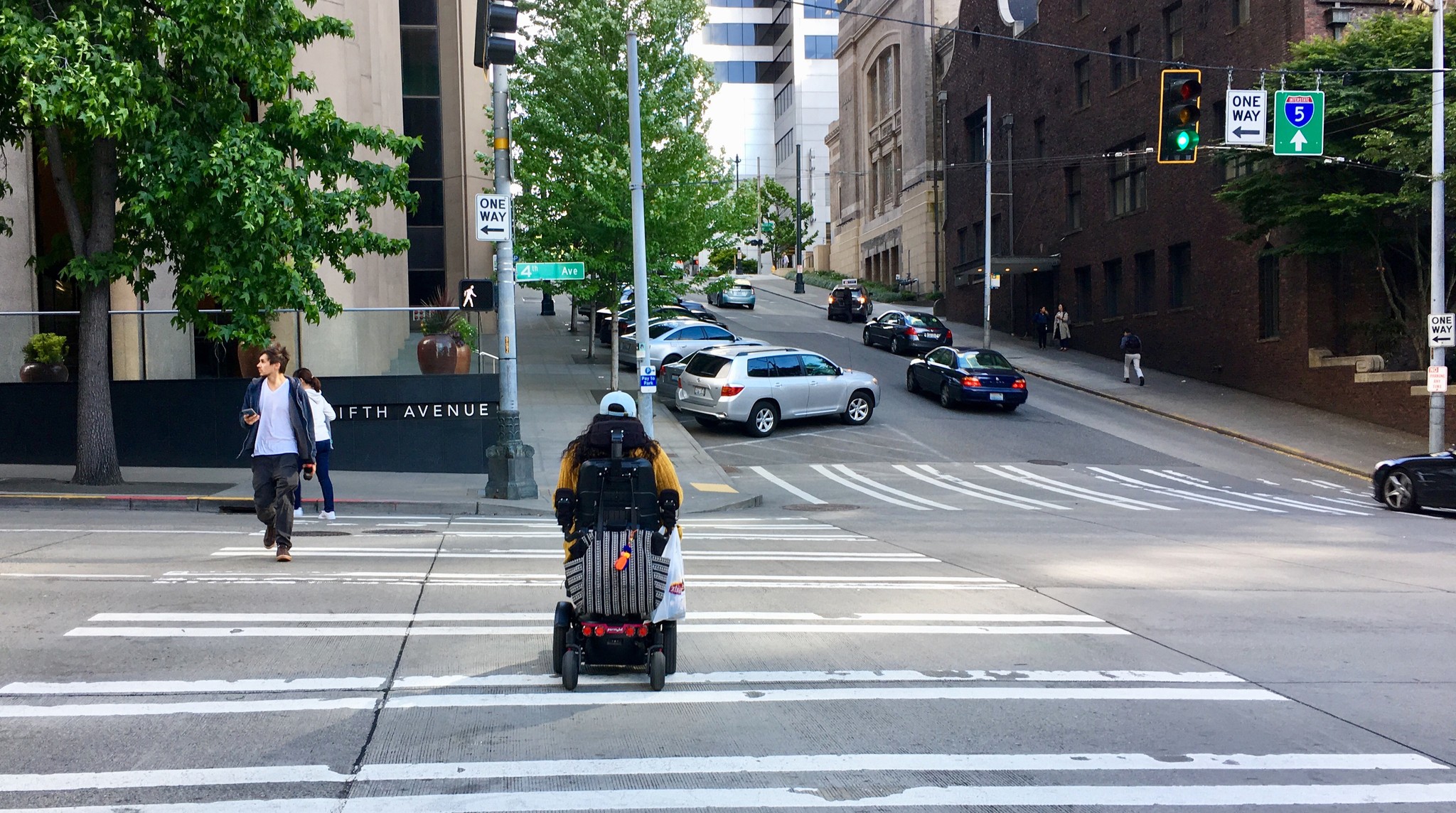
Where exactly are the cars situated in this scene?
[862,310,953,355]
[578,282,729,346]
[1369,444,1456,515]
[617,319,770,374]
[657,346,727,400]
[905,346,1029,412]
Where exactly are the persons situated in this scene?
[781,252,789,268]
[842,285,853,323]
[1030,305,1050,351]
[238,346,317,561]
[556,390,682,565]
[1054,304,1071,352]
[289,368,337,518]
[1121,328,1144,387]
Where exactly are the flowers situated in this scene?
[410,284,478,351]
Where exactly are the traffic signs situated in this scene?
[1428,313,1455,348]
[476,193,512,242]
[1224,89,1267,146]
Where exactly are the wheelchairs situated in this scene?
[552,419,679,691]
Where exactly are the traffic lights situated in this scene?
[693,260,699,265]
[459,278,494,312]
[474,0,518,69]
[751,240,763,246]
[1157,69,1203,164]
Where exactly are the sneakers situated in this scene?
[276,544,292,561]
[293,507,303,516]
[318,510,336,520]
[264,526,276,549]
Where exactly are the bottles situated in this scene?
[303,464,313,480]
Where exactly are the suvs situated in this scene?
[674,344,881,440]
[707,274,756,310]
[827,282,874,323]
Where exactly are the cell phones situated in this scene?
[241,407,258,418]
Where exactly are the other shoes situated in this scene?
[1059,348,1067,352]
[1039,348,1047,351]
[1139,378,1145,386]
[1123,380,1130,383]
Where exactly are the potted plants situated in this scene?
[18,332,71,383]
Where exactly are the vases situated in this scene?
[236,337,275,378]
[455,339,473,374]
[415,333,458,374]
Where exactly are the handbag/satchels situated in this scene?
[651,524,687,624]
[563,472,671,616]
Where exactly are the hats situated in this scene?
[599,391,637,418]
